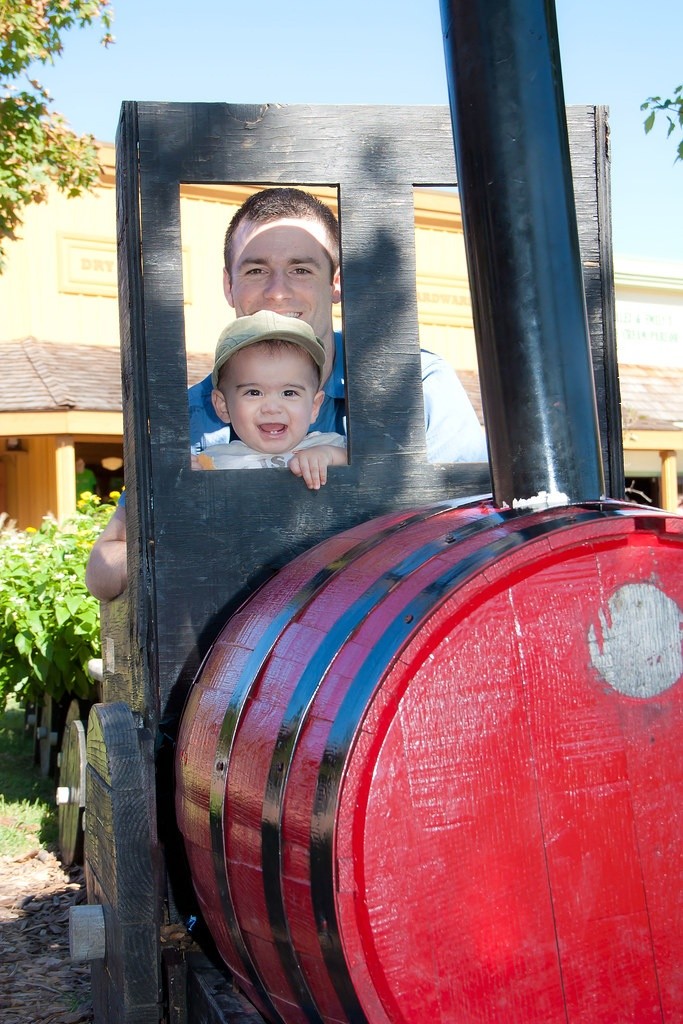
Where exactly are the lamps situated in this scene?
[5,438,27,452]
[101,457,123,470]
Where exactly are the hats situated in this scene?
[211,312,326,400]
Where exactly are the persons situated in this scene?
[85,188,490,600]
[191,309,349,490]
